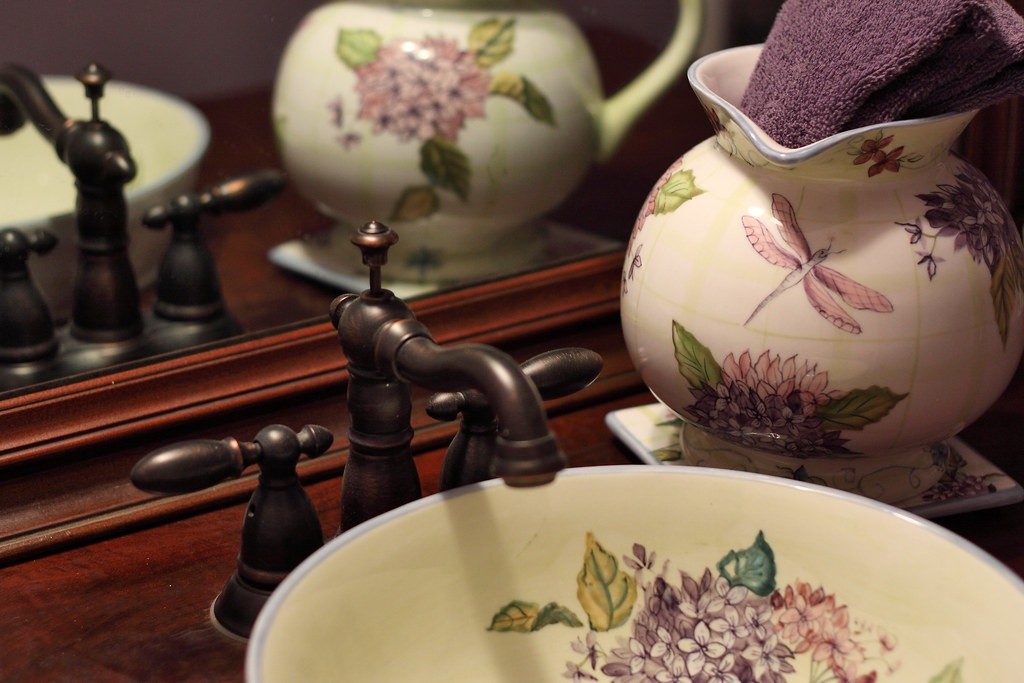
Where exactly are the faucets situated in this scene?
[329,219,568,534]
[1,60,136,342]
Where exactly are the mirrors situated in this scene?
[0,1,795,468]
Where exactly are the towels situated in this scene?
[738,0,1024,150]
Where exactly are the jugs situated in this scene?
[268,1,699,286]
[623,40,1023,507]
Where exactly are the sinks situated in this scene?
[0,70,212,318]
[244,463,1024,683]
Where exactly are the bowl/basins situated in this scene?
[0,73,212,323]
[240,465,1023,683]
